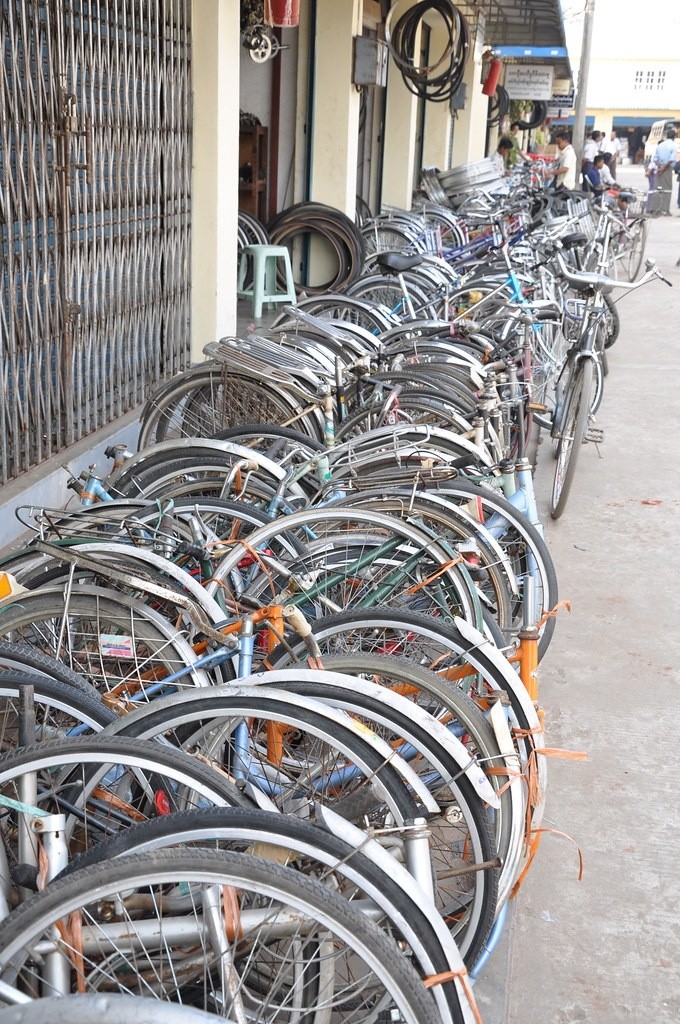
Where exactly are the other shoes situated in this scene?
[653,211,663,218]
[664,211,672,216]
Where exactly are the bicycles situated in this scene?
[0,157,673,1024]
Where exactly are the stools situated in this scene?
[237,244,297,318]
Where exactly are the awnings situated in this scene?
[613,116,675,128]
[552,116,594,128]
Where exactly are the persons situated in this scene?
[589,155,629,211]
[646,130,680,218]
[583,130,622,180]
[597,152,625,192]
[545,131,578,193]
[489,135,513,178]
[506,120,531,169]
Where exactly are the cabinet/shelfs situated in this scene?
[239,124,269,231]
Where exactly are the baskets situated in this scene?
[627,193,664,219]
